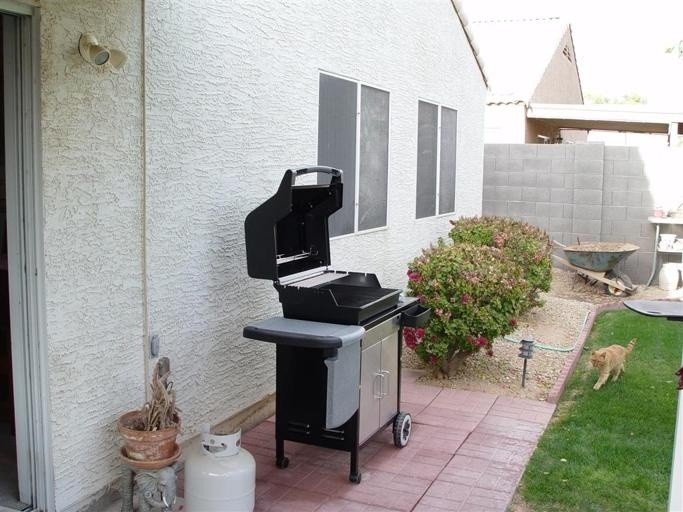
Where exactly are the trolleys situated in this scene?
[240,165,434,484]
[547,235,640,296]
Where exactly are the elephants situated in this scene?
[134,464,179,512]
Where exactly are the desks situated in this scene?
[647,217,683,287]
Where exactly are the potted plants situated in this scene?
[116,363,184,458]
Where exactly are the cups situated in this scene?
[654,209,665,218]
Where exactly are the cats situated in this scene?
[589,337,637,391]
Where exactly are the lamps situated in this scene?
[78,33,129,71]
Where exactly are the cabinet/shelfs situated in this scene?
[276,330,400,452]
